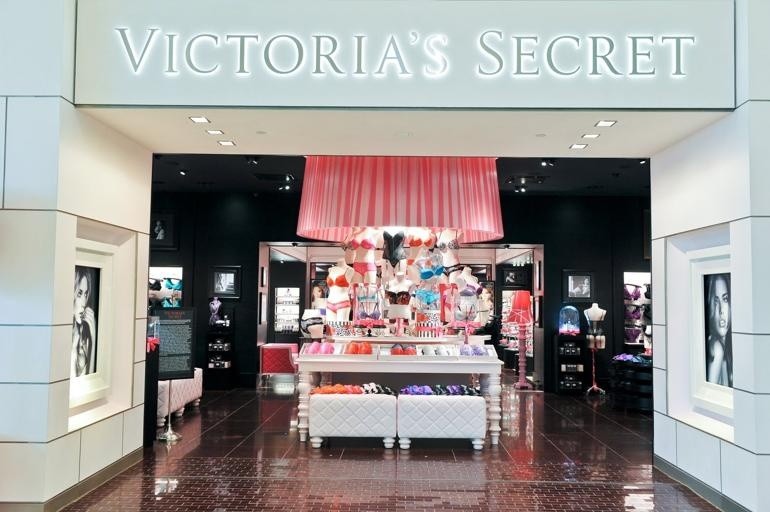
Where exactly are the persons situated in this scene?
[73,268,97,376]
[298,225,493,338]
[208,296,223,327]
[583,303,607,350]
[705,273,733,388]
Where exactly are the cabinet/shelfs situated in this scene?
[607,359,653,417]
[206,309,236,391]
[552,331,588,395]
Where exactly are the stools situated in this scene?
[307,391,487,454]
[156,366,204,428]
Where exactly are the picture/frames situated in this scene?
[213,265,243,300]
[259,266,268,325]
[502,268,528,289]
[562,268,597,304]
[643,209,651,259]
[685,243,733,415]
[66,237,120,410]
[150,204,182,252]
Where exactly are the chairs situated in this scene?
[260,342,299,389]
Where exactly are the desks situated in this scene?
[294,334,505,447]
